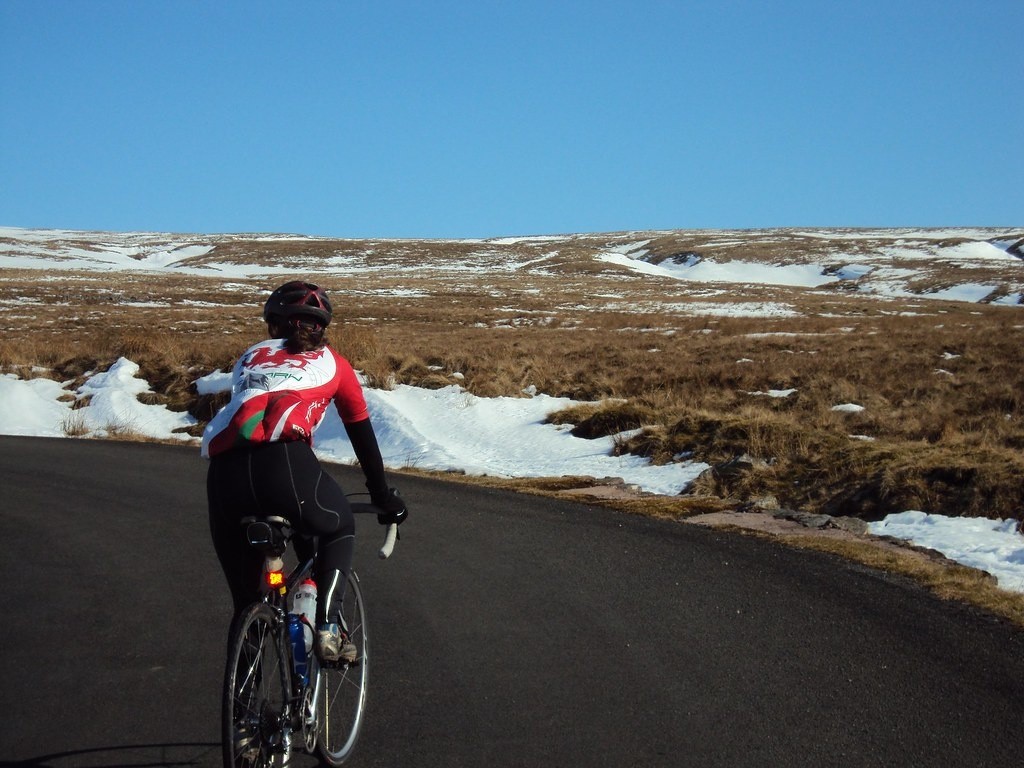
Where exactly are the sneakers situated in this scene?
[315,629,357,663]
[237,727,262,759]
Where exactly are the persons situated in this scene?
[198,281,409,752]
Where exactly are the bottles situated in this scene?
[291,579,317,653]
[287,613,309,686]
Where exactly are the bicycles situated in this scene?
[221,488,401,768]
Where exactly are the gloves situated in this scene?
[369,484,408,524]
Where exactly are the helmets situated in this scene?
[264,281,332,326]
